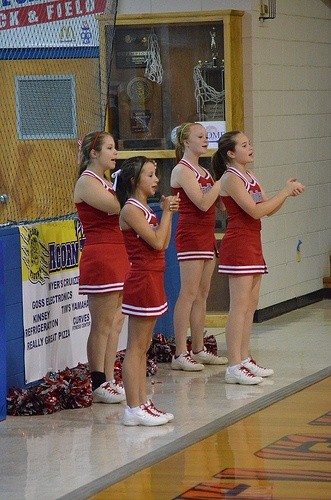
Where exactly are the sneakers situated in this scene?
[171,351,205,371]
[190,347,229,365]
[225,364,264,385]
[91,379,126,403]
[240,354,274,378]
[122,398,175,426]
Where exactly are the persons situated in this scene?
[74,131,128,403]
[108,156,181,427]
[171,123,228,371]
[212,131,306,384]
